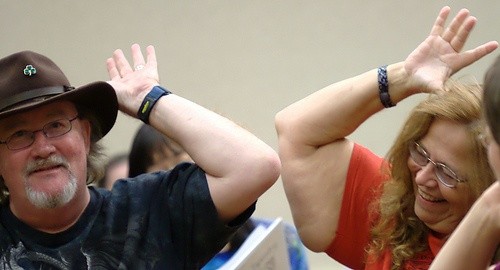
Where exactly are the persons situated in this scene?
[0,43,282,270]
[98,5,500,270]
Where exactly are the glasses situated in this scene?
[407,139,468,188]
[0,116,80,150]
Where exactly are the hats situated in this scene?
[0,50,119,144]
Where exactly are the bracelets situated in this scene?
[137,86,172,125]
[378,65,396,109]
